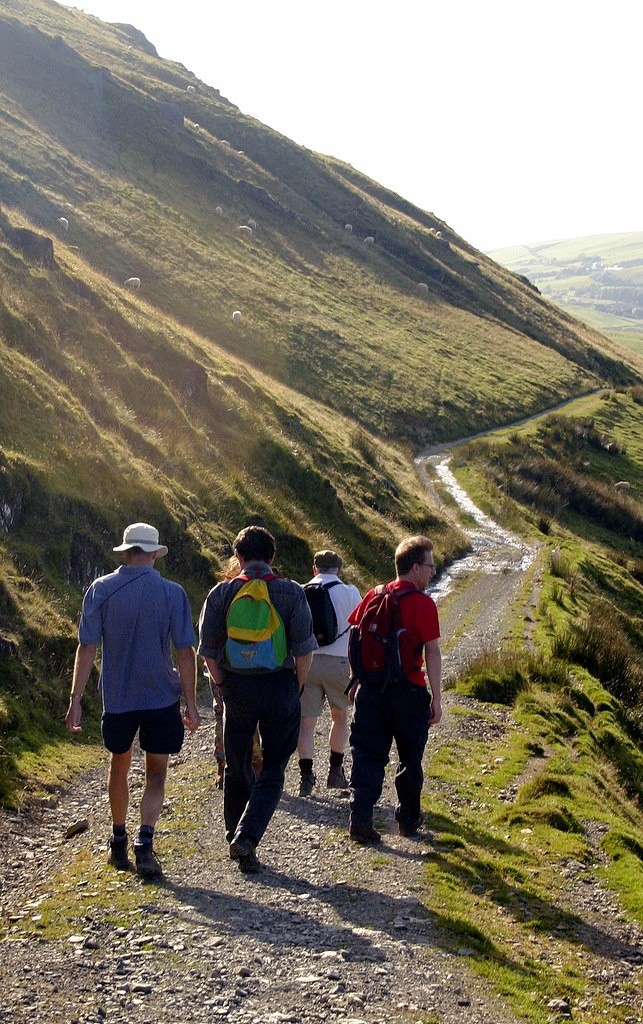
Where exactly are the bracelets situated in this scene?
[70,693,84,699]
[215,679,223,687]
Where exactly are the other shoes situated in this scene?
[298,773,314,797]
[349,825,381,843]
[231,830,259,873]
[327,767,349,788]
[224,831,235,842]
[398,812,423,836]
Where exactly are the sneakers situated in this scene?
[132,840,161,875]
[107,833,128,870]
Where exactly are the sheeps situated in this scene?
[344,224,353,232]
[239,226,252,234]
[247,219,257,229]
[364,237,374,245]
[418,283,428,290]
[123,278,141,291]
[435,232,442,238]
[430,228,436,234]
[232,311,242,320]
[216,206,222,213]
[57,217,69,232]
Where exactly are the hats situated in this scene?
[315,550,342,568]
[112,523,168,558]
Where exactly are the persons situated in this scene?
[348,535,441,840]
[298,550,362,796]
[196,526,319,875]
[65,523,201,875]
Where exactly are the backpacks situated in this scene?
[344,584,423,694]
[302,581,352,648]
[217,573,288,673]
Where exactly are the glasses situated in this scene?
[420,563,435,570]
